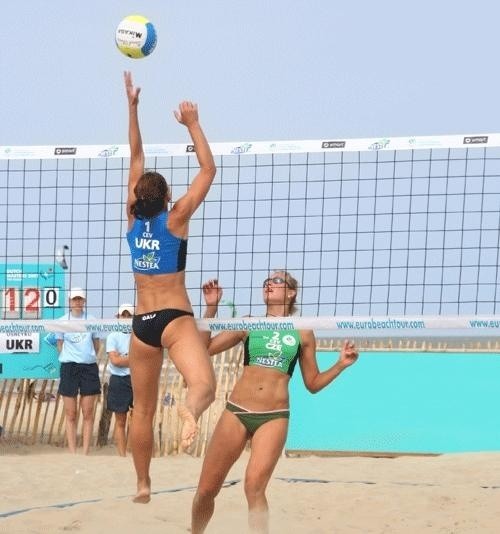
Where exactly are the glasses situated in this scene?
[262,277,291,288]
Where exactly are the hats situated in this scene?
[117,303,134,315]
[69,288,86,299]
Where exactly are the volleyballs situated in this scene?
[114,12,156,59]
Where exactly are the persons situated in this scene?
[103,304,135,454]
[55,286,101,454]
[189,270,357,533]
[121,69,218,504]
